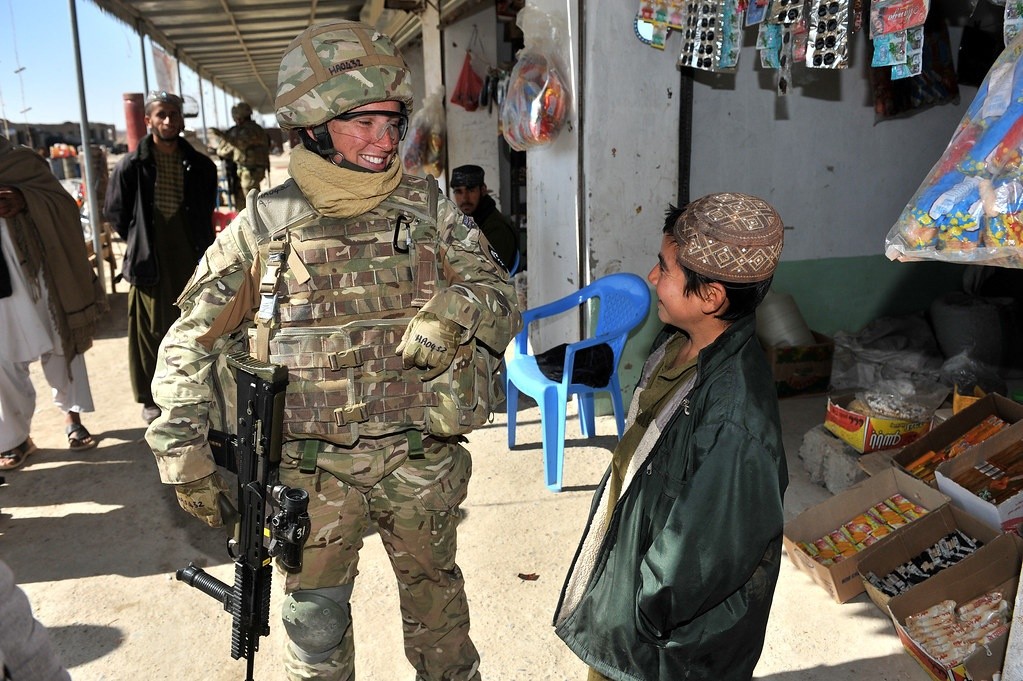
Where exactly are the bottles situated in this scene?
[755,286,815,350]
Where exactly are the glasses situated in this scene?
[335,114,407,145]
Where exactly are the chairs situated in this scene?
[506,271,651,490]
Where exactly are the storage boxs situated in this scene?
[783,390,1023,681]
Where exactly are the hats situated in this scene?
[450,165,485,188]
[672,192,784,283]
[144,90,182,115]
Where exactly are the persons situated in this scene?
[449,165,524,276]
[103,90,218,422]
[0,135,102,469]
[552,191,790,680]
[208,102,271,213]
[144,16,523,681]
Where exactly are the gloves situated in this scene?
[169,474,227,528]
[397,310,461,382]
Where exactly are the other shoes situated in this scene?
[142,404,161,424]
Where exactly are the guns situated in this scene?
[174,350,312,681]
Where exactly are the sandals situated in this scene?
[65,423,95,451]
[0,435,36,469]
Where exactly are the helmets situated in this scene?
[231,103,251,118]
[275,20,414,130]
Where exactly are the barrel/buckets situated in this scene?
[48,157,82,180]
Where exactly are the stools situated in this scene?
[213,207,237,234]
